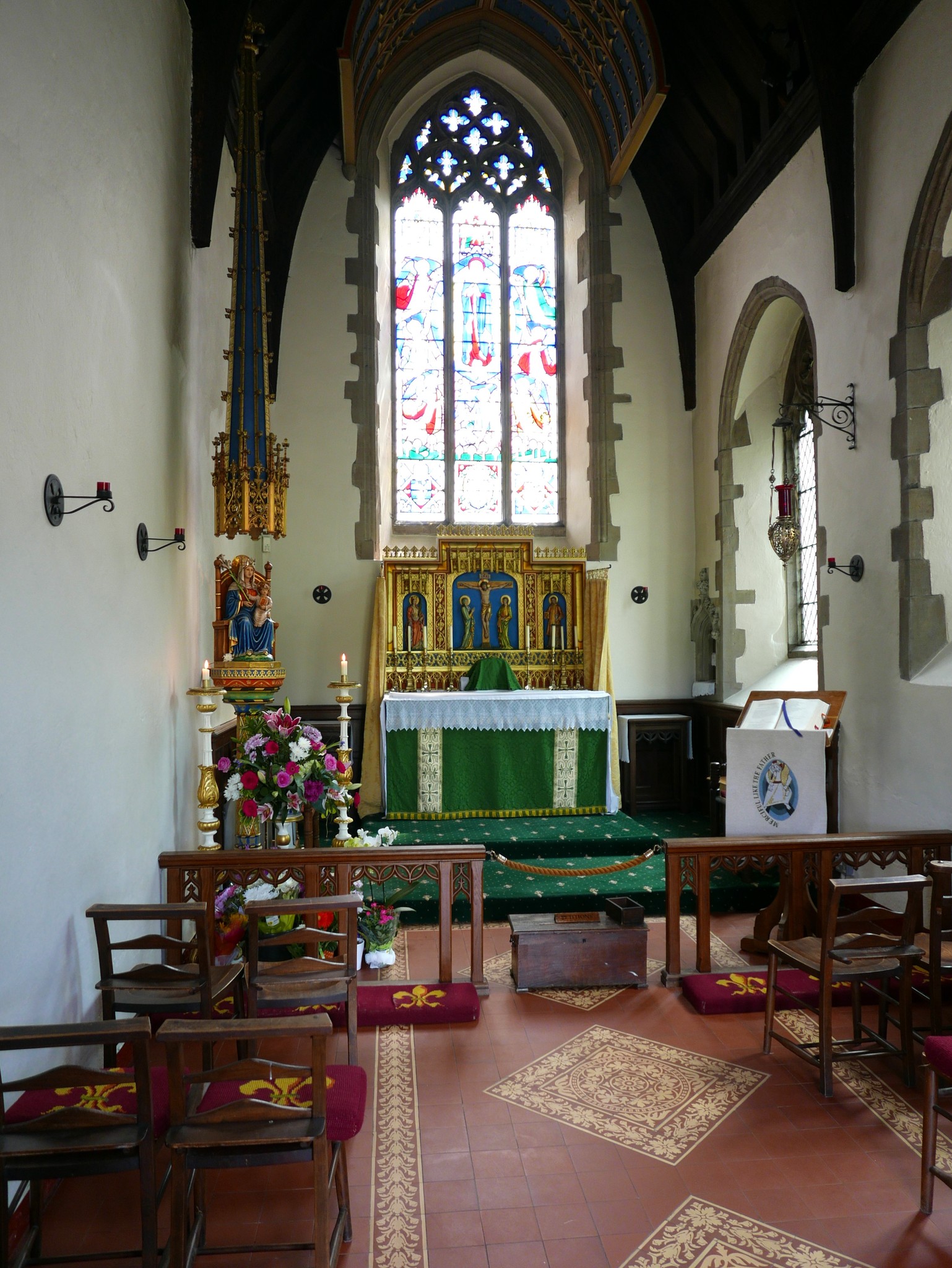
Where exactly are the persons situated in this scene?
[405,595,424,649]
[246,581,273,627]
[222,557,275,657]
[458,595,475,649]
[459,581,511,638]
[546,595,564,648]
[496,594,513,650]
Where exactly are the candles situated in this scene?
[393,626,397,648]
[828,558,836,562]
[552,625,556,647]
[574,626,578,647]
[423,626,427,649]
[526,625,530,648]
[643,587,648,590]
[175,528,185,535]
[202,660,210,680]
[408,626,411,652]
[561,626,564,651]
[97,481,111,491]
[319,586,324,590]
[341,653,348,676]
[449,625,453,648]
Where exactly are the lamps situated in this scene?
[208,15,292,540]
[767,382,856,571]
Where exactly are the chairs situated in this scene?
[86,901,244,1071]
[877,858,952,1088]
[243,891,363,1064]
[920,1035,952,1214]
[762,872,931,1100]
[153,1012,368,1268]
[0,1016,193,1268]
[707,762,728,827]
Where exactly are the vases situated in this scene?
[356,937,365,971]
[369,946,392,969]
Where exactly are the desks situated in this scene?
[291,719,355,766]
[380,689,620,821]
[617,714,695,817]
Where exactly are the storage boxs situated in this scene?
[508,911,651,992]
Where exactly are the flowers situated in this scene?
[214,696,421,961]
